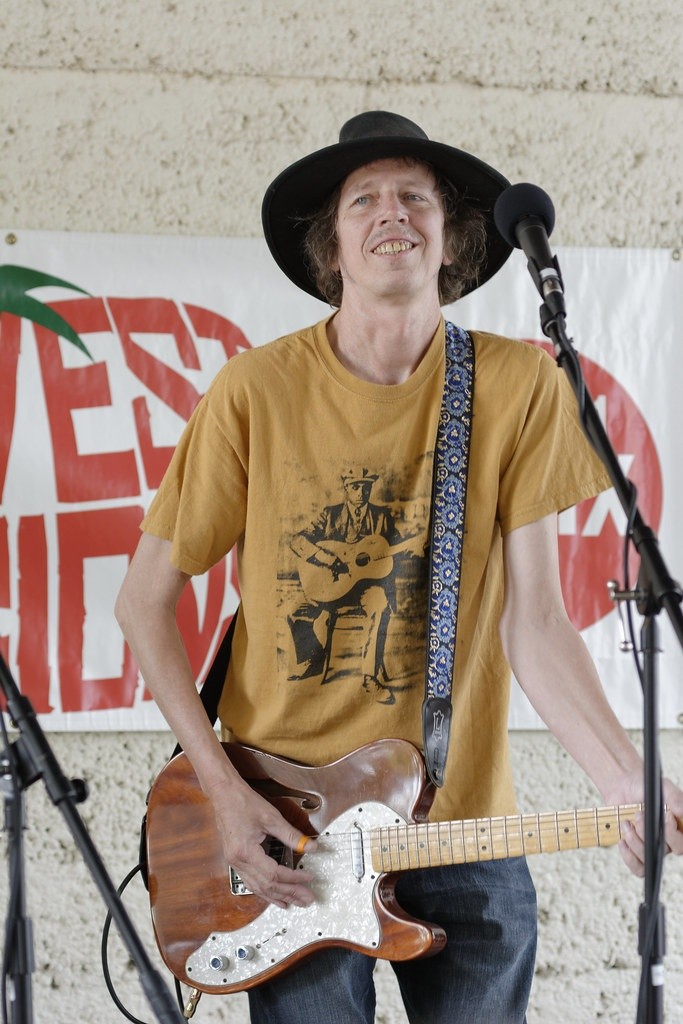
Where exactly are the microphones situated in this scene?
[493,182,567,316]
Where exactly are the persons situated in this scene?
[112,111,682,1023]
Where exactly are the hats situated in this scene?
[262,111,514,309]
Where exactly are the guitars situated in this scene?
[141,735,682,998]
